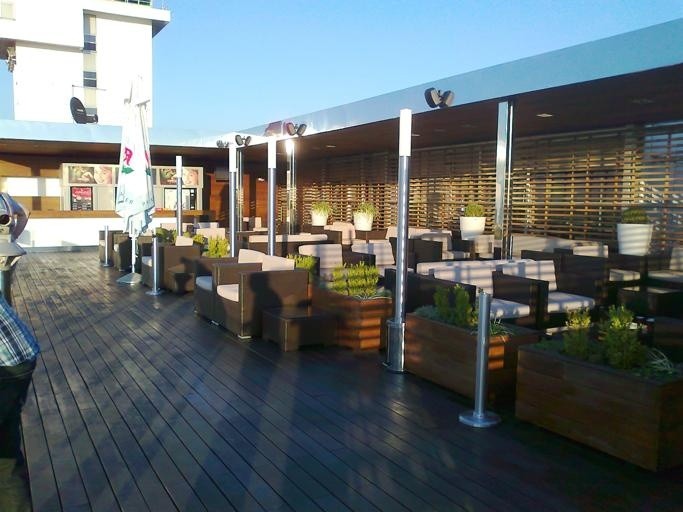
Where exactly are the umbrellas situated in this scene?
[114,72,156,273]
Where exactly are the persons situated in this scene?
[181,169,198,185]
[0,292,40,467]
[93,166,112,184]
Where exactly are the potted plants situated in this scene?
[310,202,330,226]
[617,208,654,258]
[352,202,375,231]
[459,203,487,240]
